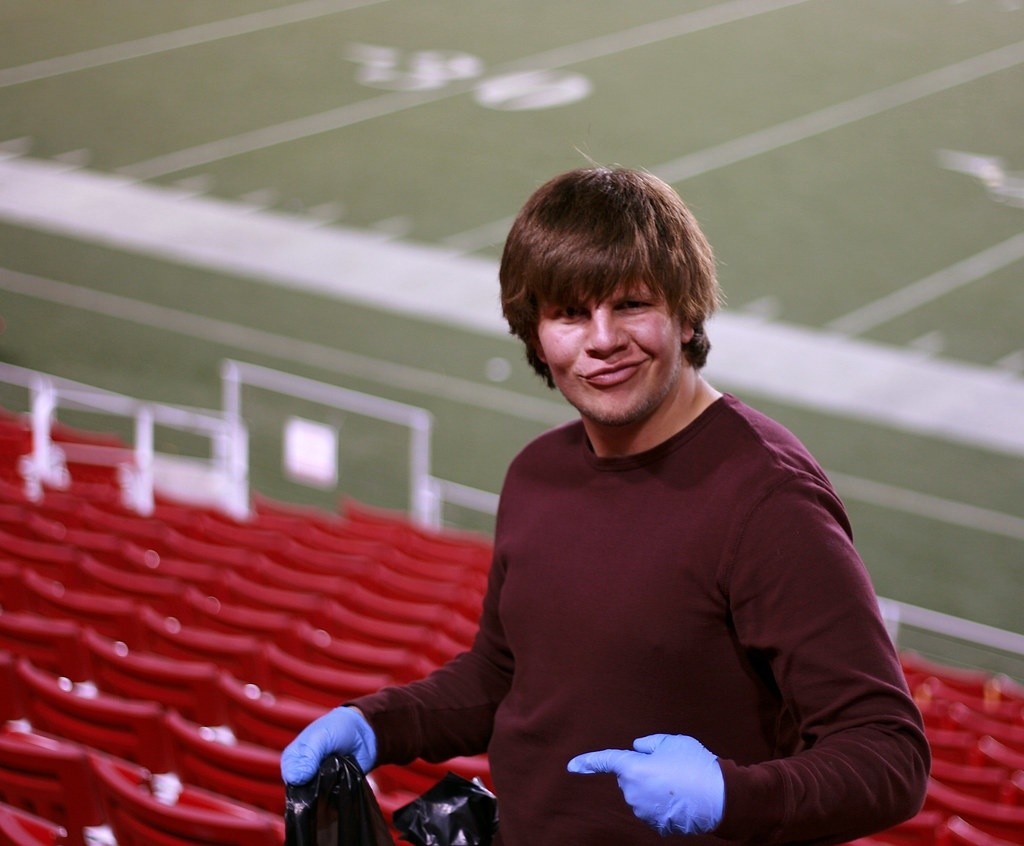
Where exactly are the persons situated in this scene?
[279,166,932,846]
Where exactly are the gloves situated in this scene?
[567,734,725,838]
[280,705,378,786]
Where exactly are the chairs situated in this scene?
[0,409,1024,846]
